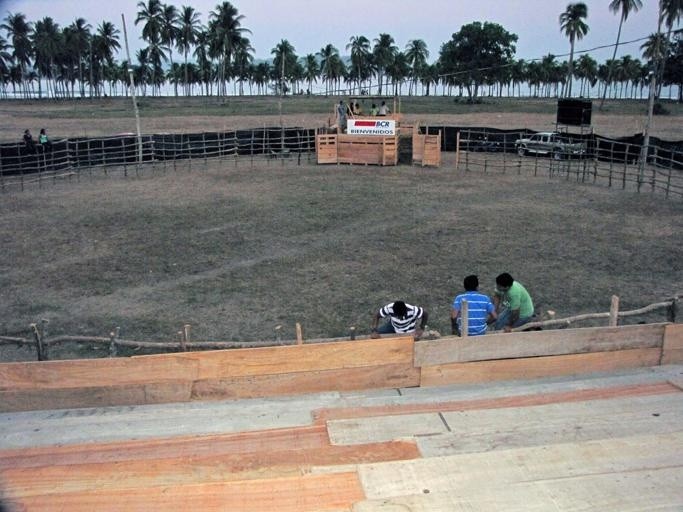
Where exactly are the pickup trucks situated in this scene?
[514,132,589,161]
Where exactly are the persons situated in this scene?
[37,128,53,153]
[369,104,380,116]
[369,299,429,340]
[338,100,346,127]
[353,104,367,117]
[346,101,354,115]
[450,274,498,337]
[22,128,36,155]
[378,100,392,115]
[282,82,288,96]
[485,272,535,333]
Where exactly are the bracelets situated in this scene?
[419,324,425,330]
[370,328,378,333]
